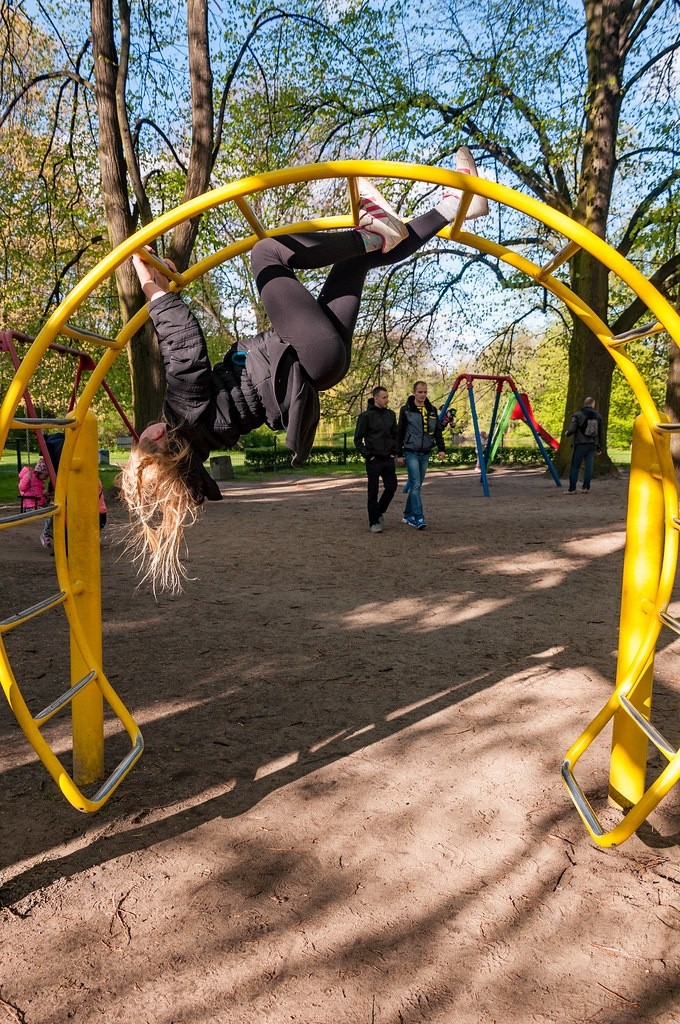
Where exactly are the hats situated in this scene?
[34,458,49,474]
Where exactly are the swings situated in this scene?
[430,381,497,450]
[16,338,52,514]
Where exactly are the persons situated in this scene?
[18,432,107,547]
[475,431,488,470]
[397,381,446,529]
[562,397,603,495]
[121,146,489,596]
[354,386,396,533]
[438,404,457,431]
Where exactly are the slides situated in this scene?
[511,393,560,450]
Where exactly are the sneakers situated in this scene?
[379,517,394,529]
[401,516,416,526]
[357,178,409,253]
[444,146,490,219]
[370,524,382,533]
[39,534,51,549]
[416,519,427,529]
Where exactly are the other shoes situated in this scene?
[580,489,589,494]
[562,491,576,494]
